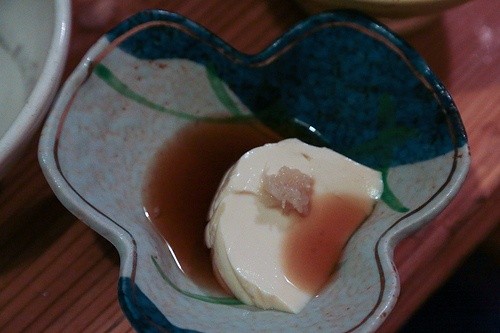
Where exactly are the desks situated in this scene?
[0,0,500,333]
